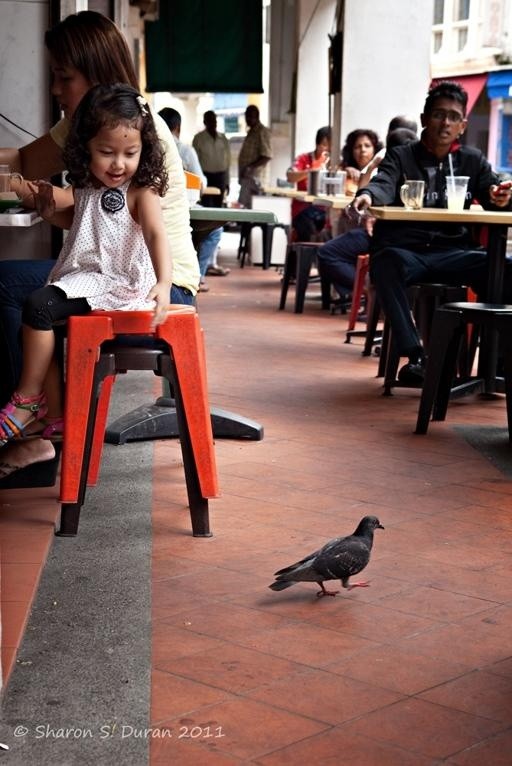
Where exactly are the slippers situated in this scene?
[0,452,58,488]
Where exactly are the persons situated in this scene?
[0,10,201,492]
[238,105,273,209]
[207,247,231,276]
[1,83,173,451]
[159,107,224,292]
[193,110,231,207]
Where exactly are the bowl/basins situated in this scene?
[310,171,347,195]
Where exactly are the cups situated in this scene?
[1,164,24,208]
[445,176,470,211]
[323,178,343,198]
[399,181,424,210]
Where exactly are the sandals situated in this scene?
[206,263,230,277]
[0,390,50,441]
[8,411,63,442]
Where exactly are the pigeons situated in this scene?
[268,515,385,598]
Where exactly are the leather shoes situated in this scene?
[398,357,425,385]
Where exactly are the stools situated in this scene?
[1,173,512,538]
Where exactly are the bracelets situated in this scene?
[12,171,24,187]
